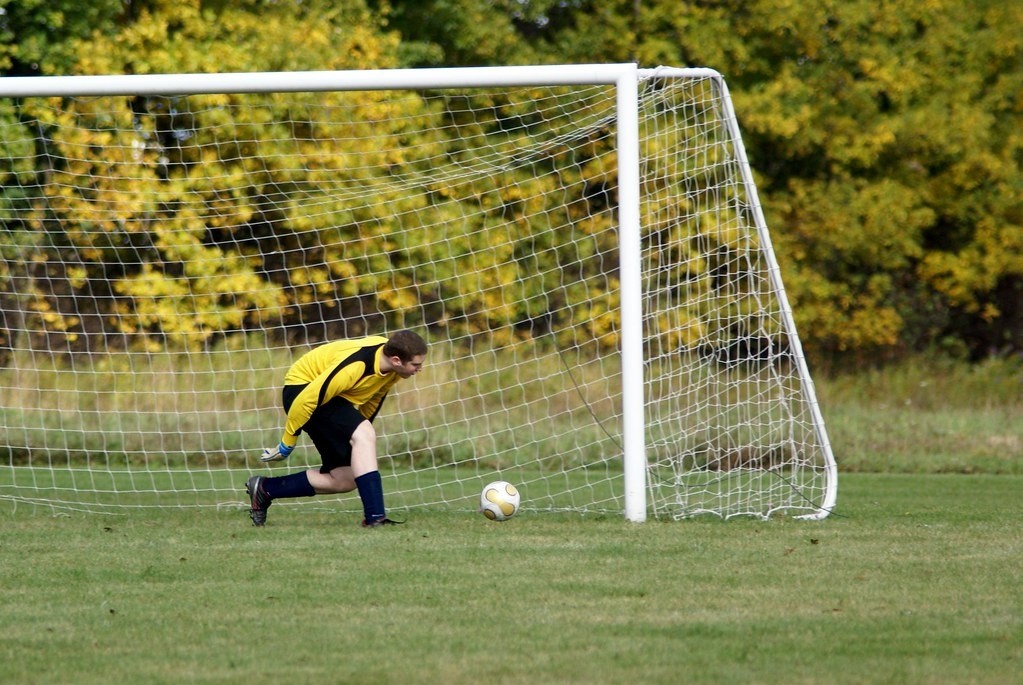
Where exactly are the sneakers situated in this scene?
[360,518,400,527]
[244,475,271,527]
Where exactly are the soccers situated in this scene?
[479,480,521,523]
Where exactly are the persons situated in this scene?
[244,329,428,528]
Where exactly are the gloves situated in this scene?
[260,442,294,462]
